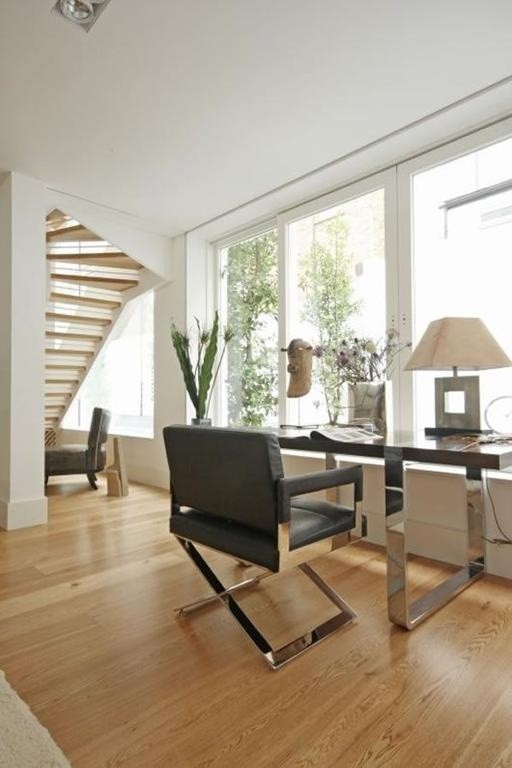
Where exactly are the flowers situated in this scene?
[306,330,410,380]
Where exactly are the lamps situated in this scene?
[409,315,511,428]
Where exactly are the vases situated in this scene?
[346,383,387,426]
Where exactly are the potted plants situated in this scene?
[171,310,237,427]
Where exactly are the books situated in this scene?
[265,427,384,444]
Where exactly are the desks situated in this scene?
[236,427,512,632]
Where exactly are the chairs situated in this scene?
[45,408,112,491]
[162,425,370,669]
